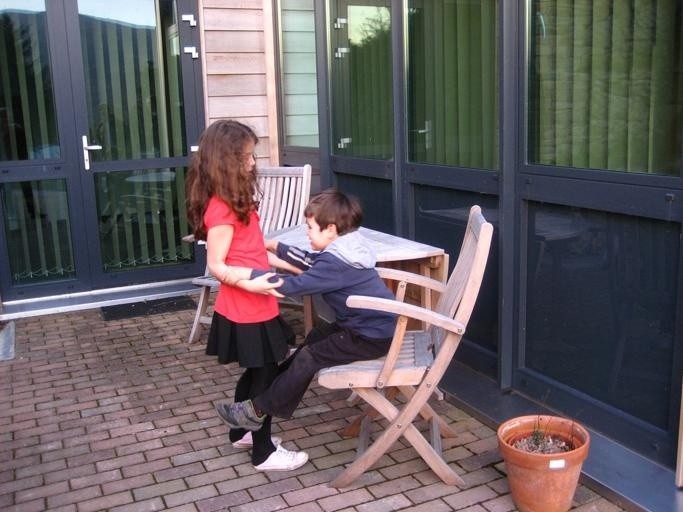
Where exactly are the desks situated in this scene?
[262,223,450,380]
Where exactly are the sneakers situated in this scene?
[252,445,310,472]
[229,431,283,449]
[215,397,267,434]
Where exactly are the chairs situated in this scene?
[182,164,312,345]
[316,205,495,489]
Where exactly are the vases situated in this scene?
[496,415,590,512]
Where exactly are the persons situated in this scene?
[214,186,397,432]
[180,120,310,472]
